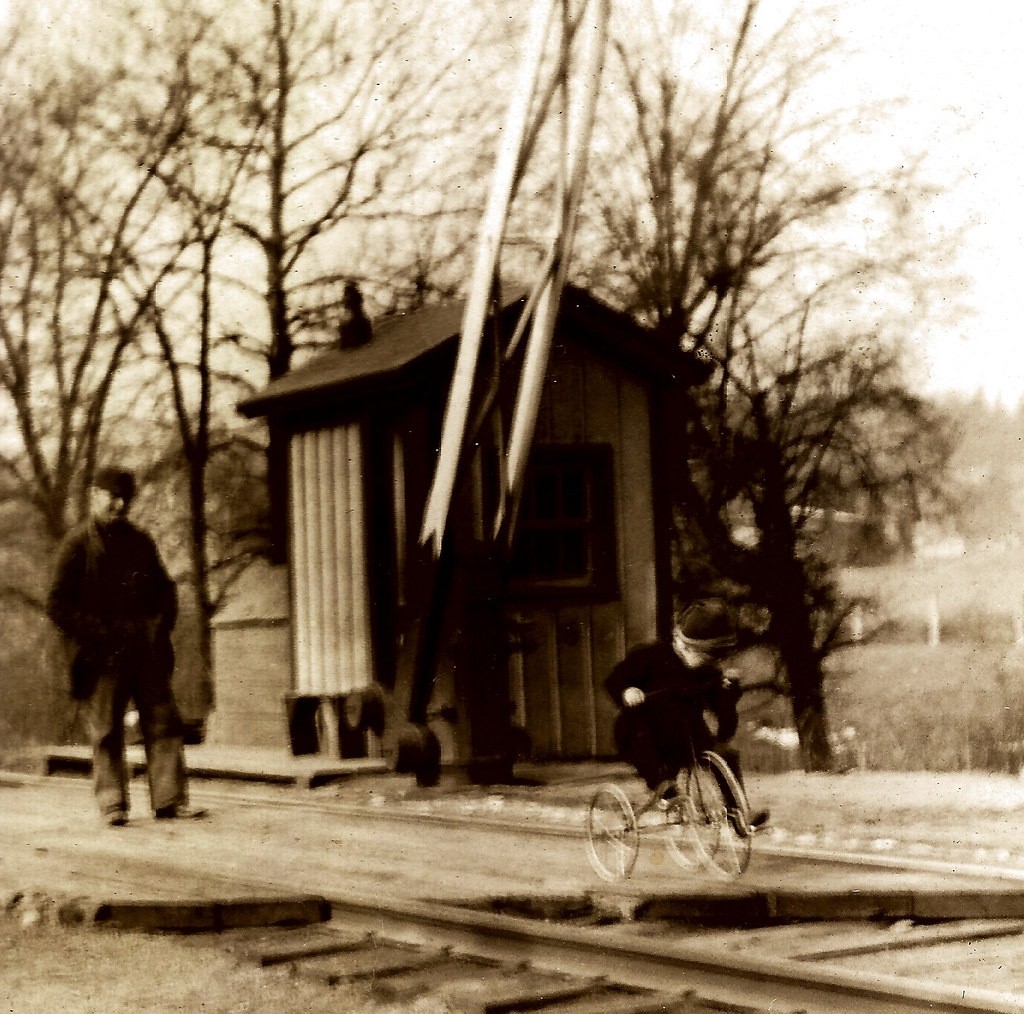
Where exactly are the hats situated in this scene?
[675,598,738,647]
[92,465,137,495]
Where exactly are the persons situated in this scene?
[601,598,770,838]
[46,466,207,824]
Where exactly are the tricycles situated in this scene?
[581,688,773,886]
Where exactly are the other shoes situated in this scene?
[660,781,677,800]
[104,810,129,824]
[156,805,204,817]
[750,812,768,826]
